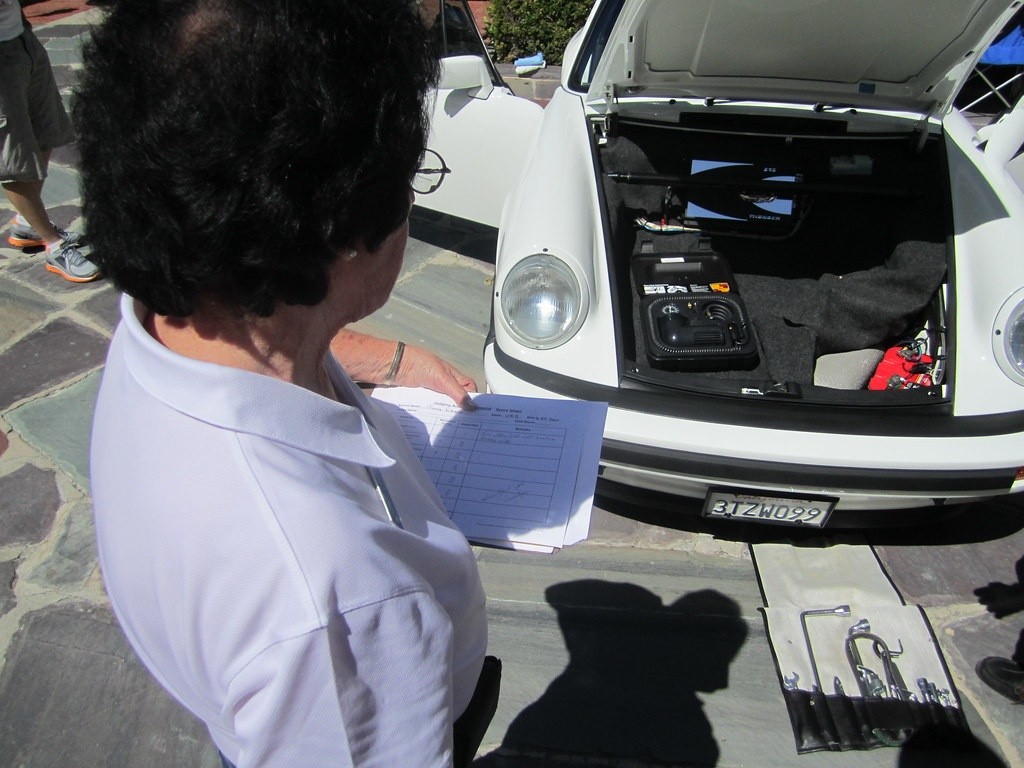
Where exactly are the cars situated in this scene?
[408,0,1023,528]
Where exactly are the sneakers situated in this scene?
[8,214,81,247]
[45,240,102,282]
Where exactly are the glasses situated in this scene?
[408,148,452,195]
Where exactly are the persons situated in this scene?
[56,0,489,768]
[973,654,1024,711]
[0,0,101,282]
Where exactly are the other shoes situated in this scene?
[975,656,1024,704]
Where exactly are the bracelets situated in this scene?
[384,341,404,387]
